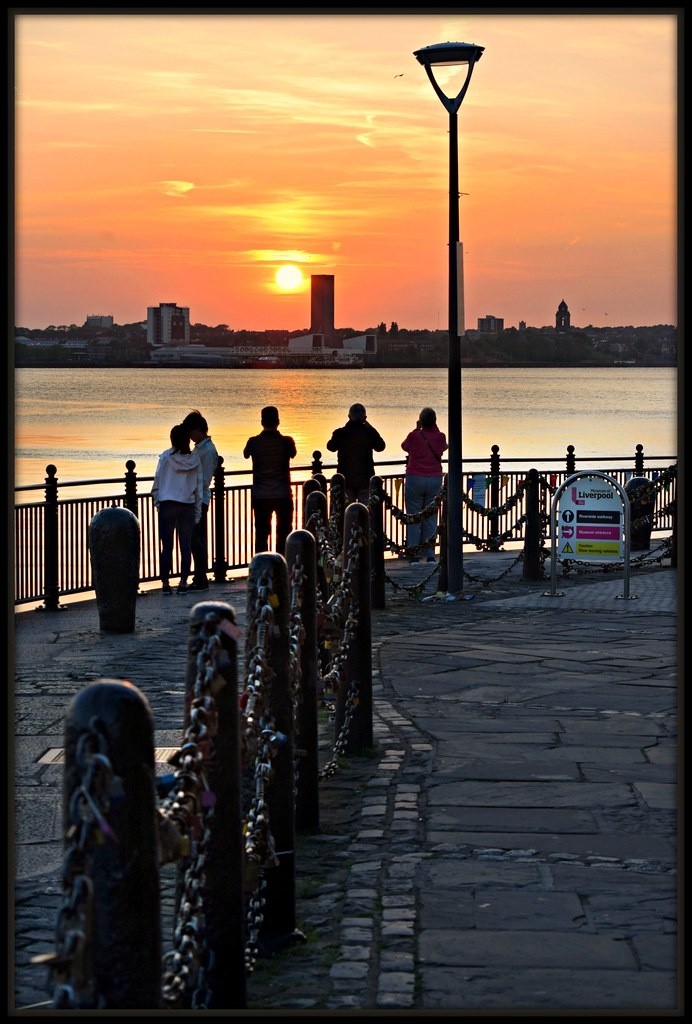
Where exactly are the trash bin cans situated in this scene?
[620,477,657,551]
[86,505,141,634]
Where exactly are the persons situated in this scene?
[326,403,386,507]
[401,407,448,566]
[243,406,296,560]
[182,409,219,592]
[151,424,203,595]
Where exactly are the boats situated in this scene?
[247,356,287,368]
[305,350,364,368]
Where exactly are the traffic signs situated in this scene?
[554,477,624,565]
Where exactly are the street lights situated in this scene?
[411,41,488,593]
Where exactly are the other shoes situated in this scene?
[408,557,420,565]
[426,557,436,564]
[188,580,209,591]
[176,586,188,594]
[162,586,173,594]
